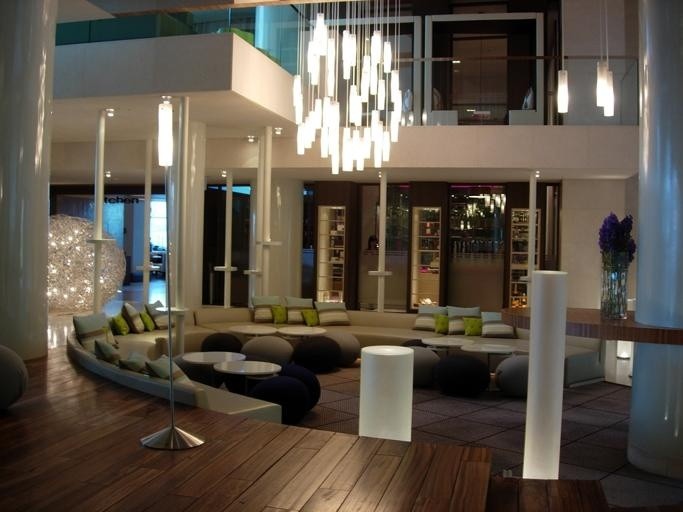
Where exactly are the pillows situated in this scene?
[412,302,514,339]
[249,294,351,329]
[72,296,193,387]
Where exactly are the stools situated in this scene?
[400,338,529,399]
[177,328,359,425]
[0,344,29,407]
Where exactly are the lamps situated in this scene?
[518,264,567,482]
[291,0,408,173]
[138,99,206,454]
[551,1,617,118]
[357,343,413,445]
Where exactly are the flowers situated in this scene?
[598,210,636,315]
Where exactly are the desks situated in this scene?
[500,303,682,346]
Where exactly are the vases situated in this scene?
[600,251,630,319]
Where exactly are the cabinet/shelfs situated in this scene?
[408,205,443,307]
[510,208,540,312]
[315,205,346,302]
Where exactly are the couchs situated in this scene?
[65,293,606,426]
